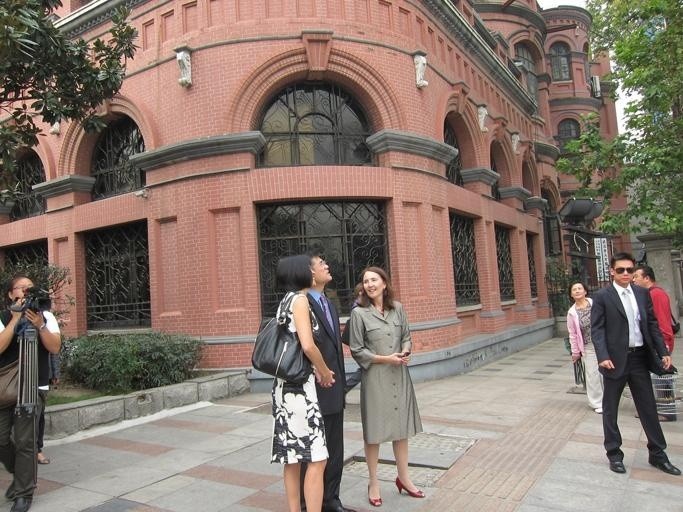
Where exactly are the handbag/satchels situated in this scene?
[670,320,680,334]
[251,293,313,385]
[342,306,357,345]
[1,358,20,410]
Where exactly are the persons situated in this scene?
[271,256,337,512]
[591,251,681,476]
[350,266,425,507]
[567,281,605,415]
[341,282,363,394]
[301,249,355,512]
[37,351,60,464]
[0,276,61,512]
[632,264,677,421]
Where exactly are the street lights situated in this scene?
[557,195,605,291]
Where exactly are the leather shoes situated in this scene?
[594,408,602,414]
[609,461,626,473]
[10,497,32,512]
[6,480,17,498]
[336,504,355,512]
[655,462,680,475]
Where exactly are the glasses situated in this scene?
[614,267,633,275]
[12,284,32,291]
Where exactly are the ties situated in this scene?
[320,295,330,321]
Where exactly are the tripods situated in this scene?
[15,327,39,419]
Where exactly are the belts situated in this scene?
[629,346,644,352]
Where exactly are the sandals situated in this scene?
[38,457,51,464]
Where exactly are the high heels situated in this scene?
[396,477,425,497]
[367,482,382,506]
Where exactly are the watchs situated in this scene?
[39,324,46,331]
